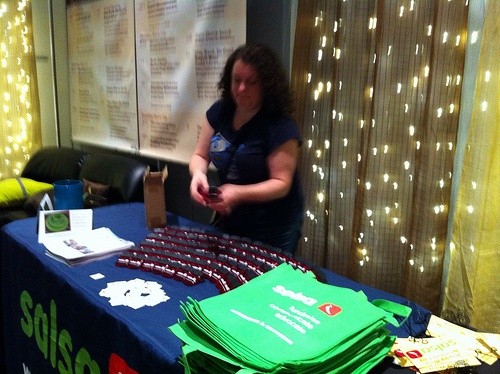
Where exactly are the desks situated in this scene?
[0,203,500,374]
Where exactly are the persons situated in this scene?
[190,44,305,255]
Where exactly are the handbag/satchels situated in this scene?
[168,263,413,374]
[209,210,221,227]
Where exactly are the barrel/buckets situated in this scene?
[54,180,83,210]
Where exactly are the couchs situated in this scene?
[0,147,223,226]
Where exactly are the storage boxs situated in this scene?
[143,165,168,230]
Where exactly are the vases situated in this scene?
[54,180,84,210]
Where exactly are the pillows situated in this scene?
[0,177,54,209]
[83,178,108,208]
